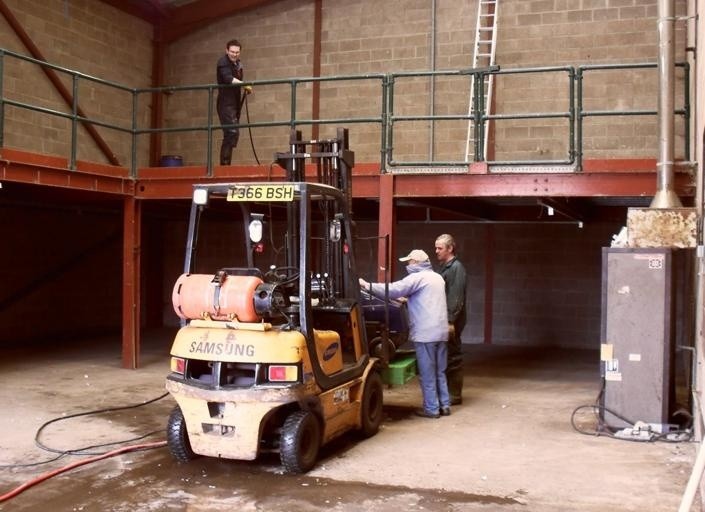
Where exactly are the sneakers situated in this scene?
[440,408,449,416]
[415,409,439,418]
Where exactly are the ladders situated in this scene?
[465,0,498,162]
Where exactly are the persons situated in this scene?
[358,248,451,418]
[435,233,465,405]
[215,40,252,165]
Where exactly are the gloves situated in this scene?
[242,85,253,93]
[449,324,455,338]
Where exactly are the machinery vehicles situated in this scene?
[166,124,421,480]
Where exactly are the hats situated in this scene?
[399,249,429,263]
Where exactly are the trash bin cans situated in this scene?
[160,154,183,167]
